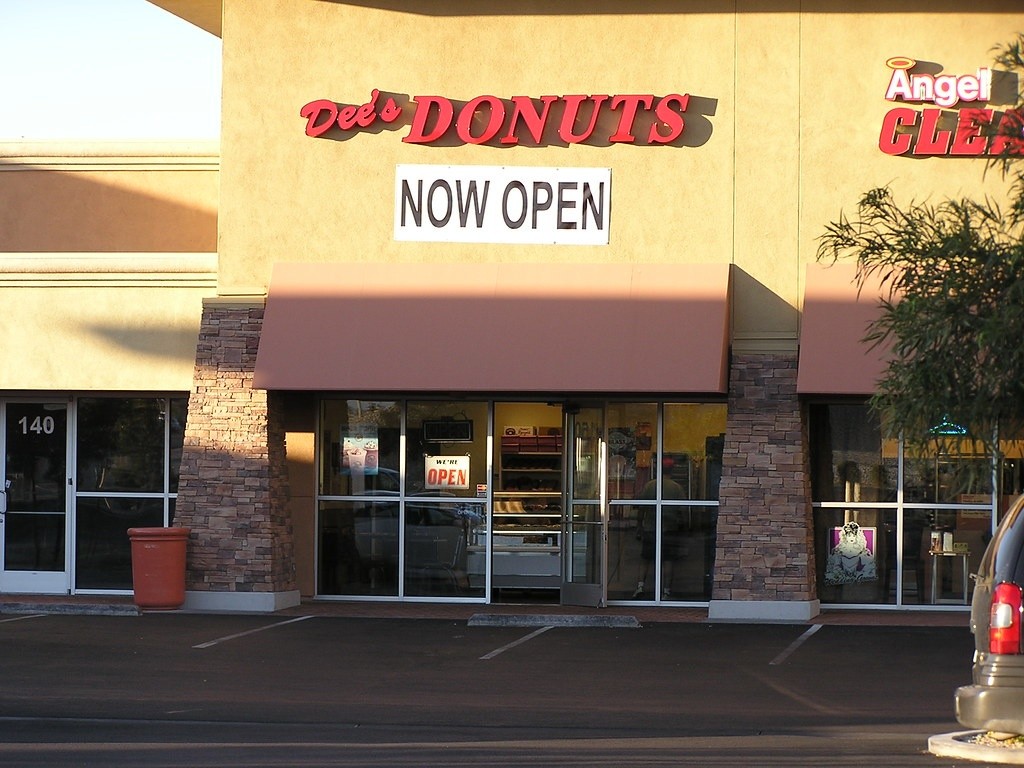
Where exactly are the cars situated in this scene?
[954,484,1024,737]
[321,460,480,604]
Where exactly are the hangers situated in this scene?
[926,413,966,435]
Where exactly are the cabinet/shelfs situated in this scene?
[493,452,562,546]
[650,454,694,530]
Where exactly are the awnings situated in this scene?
[796,264,999,397]
[254,261,730,390]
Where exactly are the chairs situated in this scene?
[883,526,925,604]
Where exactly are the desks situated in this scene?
[928,550,971,605]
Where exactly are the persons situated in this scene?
[634,459,685,599]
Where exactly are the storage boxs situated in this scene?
[519,436,537,444]
[538,444,557,452]
[501,435,519,444]
[556,435,562,444]
[956,494,992,530]
[538,436,556,444]
[520,444,537,451]
[557,444,562,452]
[502,444,519,451]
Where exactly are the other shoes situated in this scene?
[632,587,645,599]
[662,592,671,600]
